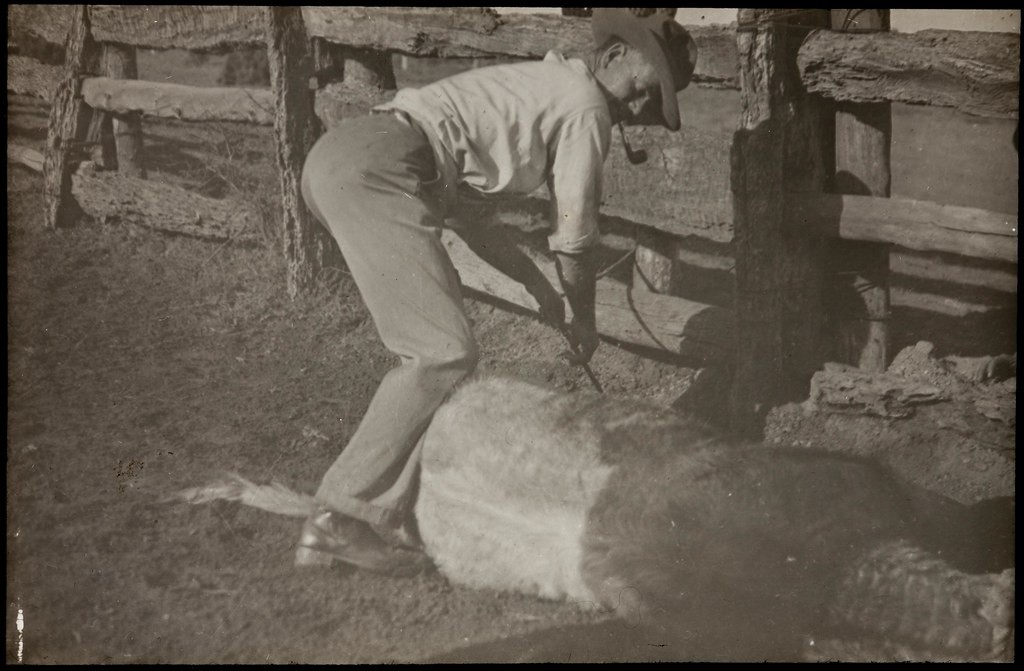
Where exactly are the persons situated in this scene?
[292,8,698,576]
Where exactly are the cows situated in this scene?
[175,371,1015,666]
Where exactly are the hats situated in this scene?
[592,7,698,131]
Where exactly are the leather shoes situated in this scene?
[294,504,423,577]
